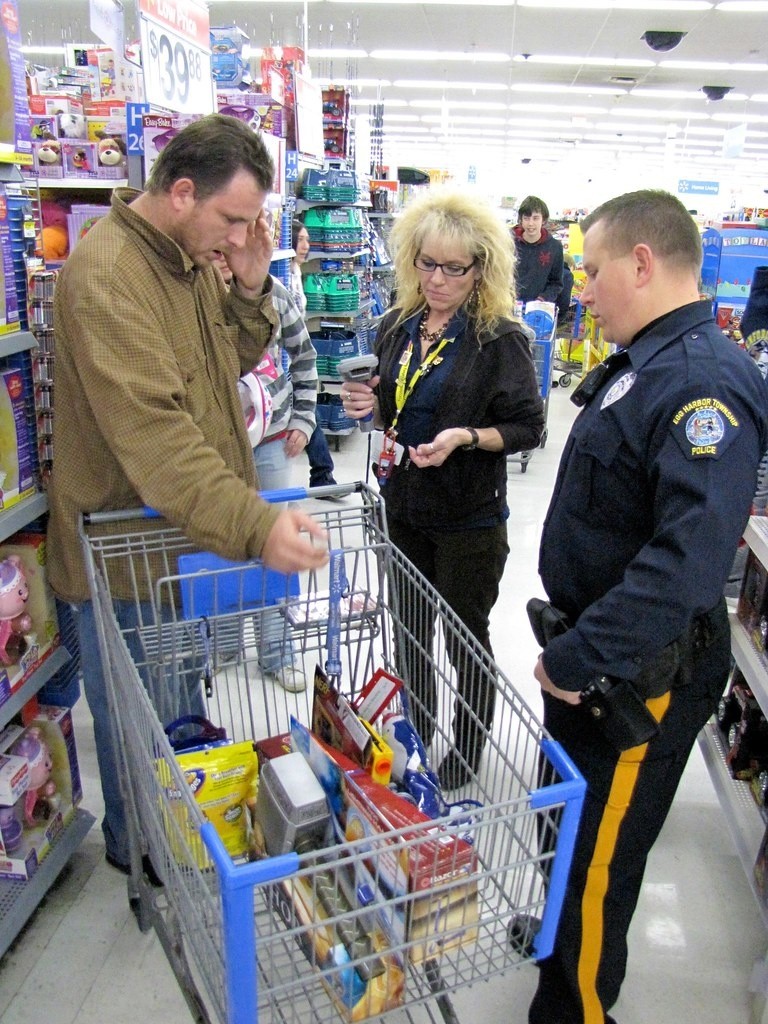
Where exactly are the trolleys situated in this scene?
[504,301,560,474]
[70,474,593,1024]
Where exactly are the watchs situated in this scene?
[462,426,479,450]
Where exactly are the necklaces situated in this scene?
[420,308,453,341]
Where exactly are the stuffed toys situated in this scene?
[59,114,83,137]
[23,188,70,260]
[40,133,62,166]
[95,130,128,168]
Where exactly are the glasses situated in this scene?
[413,249,478,277]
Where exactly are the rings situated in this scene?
[290,439,295,445]
[346,392,350,400]
[430,444,434,452]
[427,455,430,465]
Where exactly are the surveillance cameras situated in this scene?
[763,189,768,194]
[701,85,732,100]
[643,30,685,52]
[616,133,624,137]
[522,158,531,164]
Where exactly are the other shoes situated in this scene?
[506,917,541,960]
[438,740,483,790]
[105,852,164,888]
[417,716,439,746]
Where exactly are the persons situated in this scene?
[554,261,574,328]
[507,190,768,1024]
[739,266,768,383]
[289,221,351,499]
[340,194,545,791]
[514,195,563,389]
[101,59,115,90]
[43,115,330,886]
[200,252,318,690]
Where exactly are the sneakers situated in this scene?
[198,648,237,680]
[276,661,306,693]
